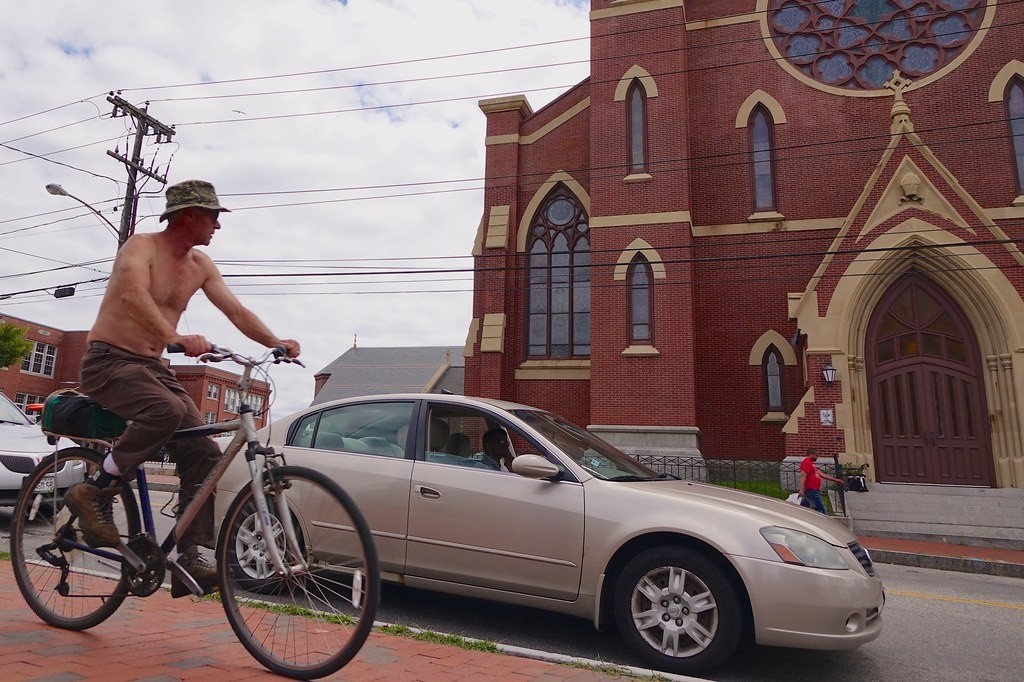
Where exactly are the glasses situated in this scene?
[498,439,510,445]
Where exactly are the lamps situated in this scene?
[822,363,836,387]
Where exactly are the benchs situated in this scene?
[312,431,406,458]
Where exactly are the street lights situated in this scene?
[46,184,134,251]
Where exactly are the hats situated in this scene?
[159,181,231,220]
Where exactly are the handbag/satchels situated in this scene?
[785,493,803,505]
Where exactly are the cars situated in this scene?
[0,389,88,517]
[178,392,885,677]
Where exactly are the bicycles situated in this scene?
[10,343,381,680]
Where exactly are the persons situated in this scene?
[474,428,510,473]
[799,448,844,514]
[66,180,302,599]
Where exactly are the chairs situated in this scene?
[429,418,451,452]
[447,432,472,457]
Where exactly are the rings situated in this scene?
[296,350,300,354]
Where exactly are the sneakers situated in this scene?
[64,483,122,547]
[172,554,217,598]
